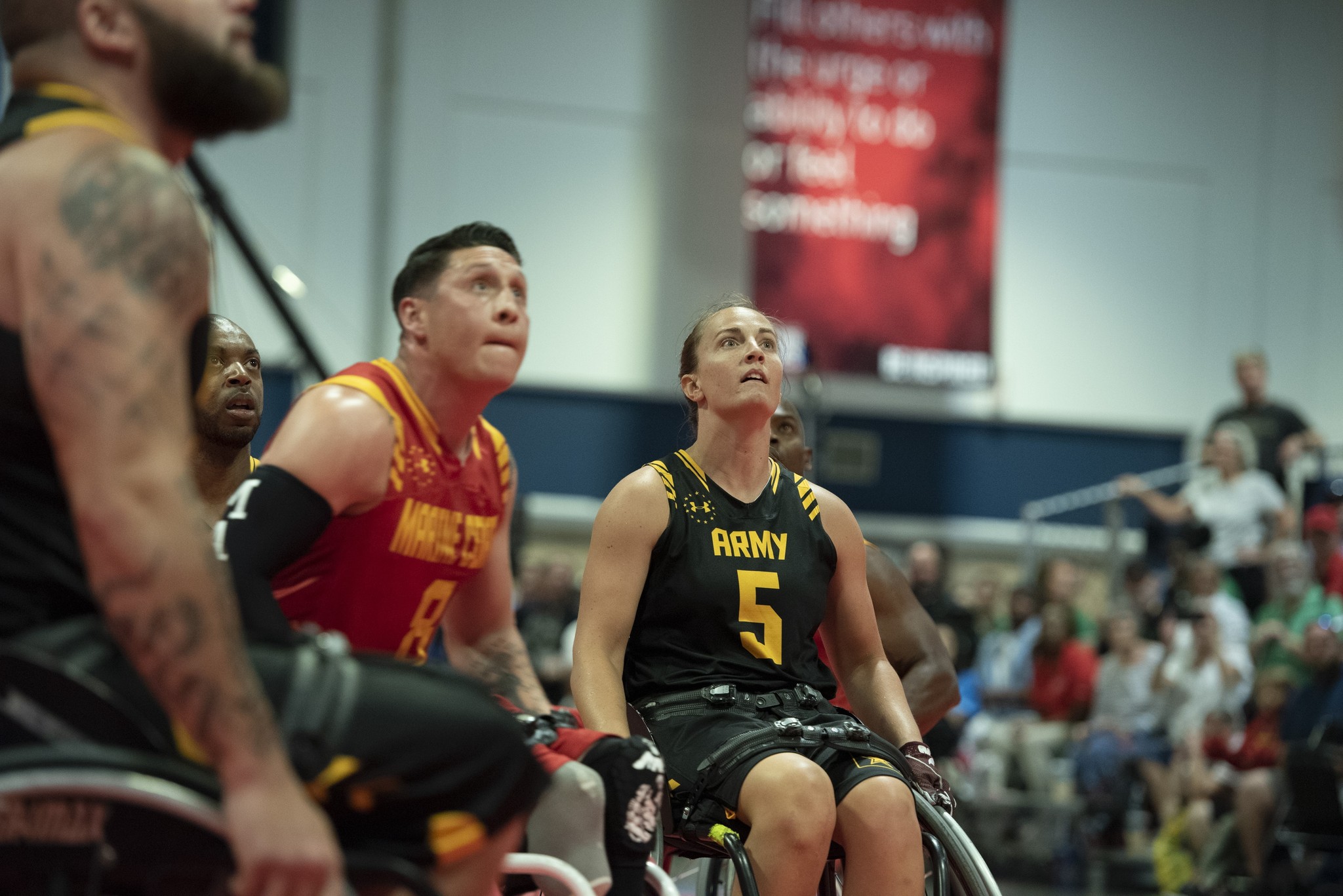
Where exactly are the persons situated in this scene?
[192,310,265,564]
[513,350,1342,896]
[572,303,939,895]
[216,221,666,895]
[0,1,345,896]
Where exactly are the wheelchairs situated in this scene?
[623,684,1003,896]
[0,614,685,896]
